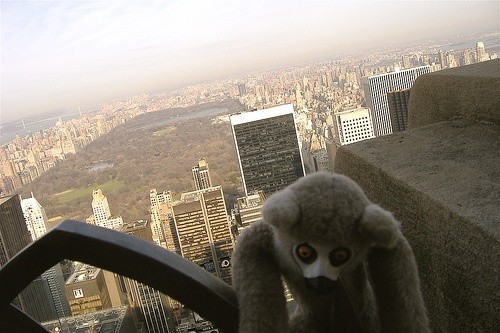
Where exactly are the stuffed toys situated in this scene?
[229,171,430,333]
